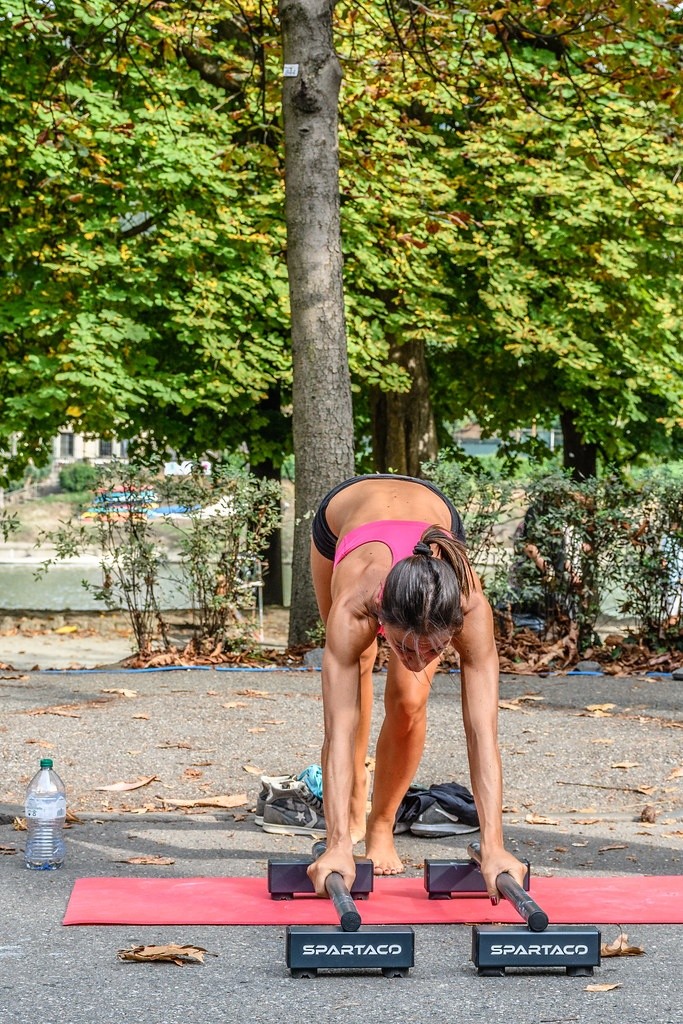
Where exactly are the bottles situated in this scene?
[25,759,66,870]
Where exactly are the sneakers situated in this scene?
[263,780,327,838]
[255,775,301,827]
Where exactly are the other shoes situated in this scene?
[409,800,479,837]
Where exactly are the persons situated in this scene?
[658,523,683,628]
[306,473,528,906]
[514,519,552,581]
